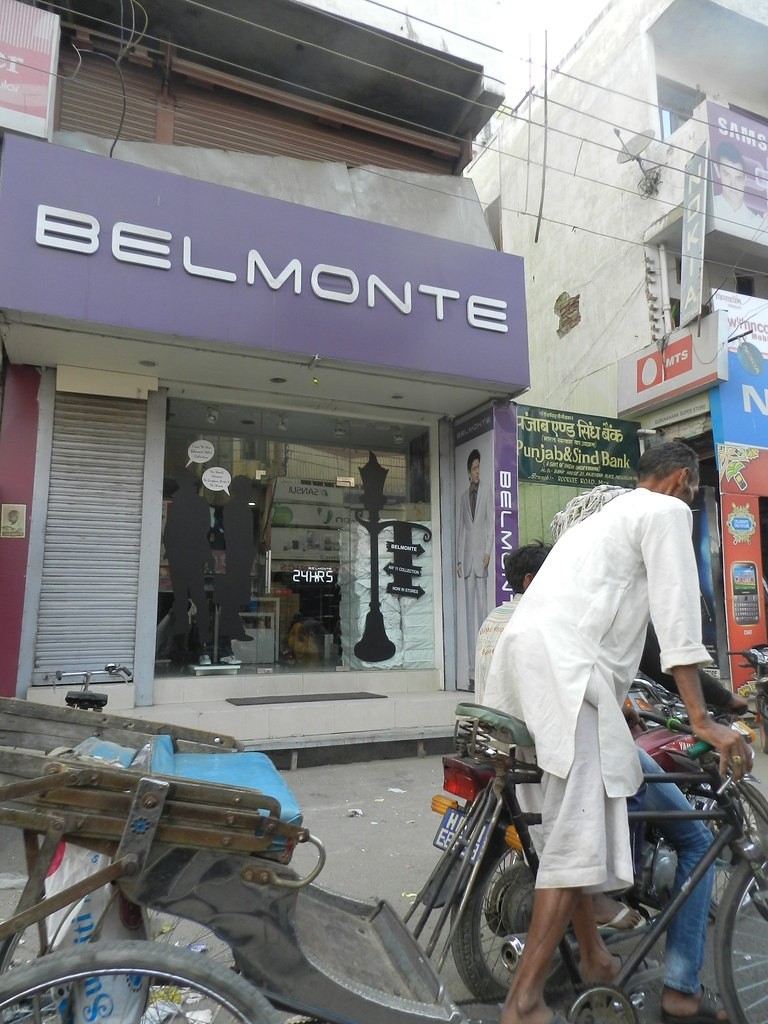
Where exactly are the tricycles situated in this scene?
[2,702,768,1024]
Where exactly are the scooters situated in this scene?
[726,644,768,753]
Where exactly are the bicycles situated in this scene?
[56,662,133,722]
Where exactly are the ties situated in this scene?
[471,489,477,520]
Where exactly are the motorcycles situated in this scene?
[427,670,768,1004]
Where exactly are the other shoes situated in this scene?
[468,679,475,691]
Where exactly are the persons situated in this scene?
[480,440,754,1023]
[456,450,493,691]
[287,611,326,671]
[713,141,768,246]
[474,538,648,932]
[198,631,243,666]
[620,622,748,1023]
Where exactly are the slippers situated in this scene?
[661,984,728,1024]
[596,901,646,931]
[578,953,666,986]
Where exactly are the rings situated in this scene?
[732,756,742,763]
